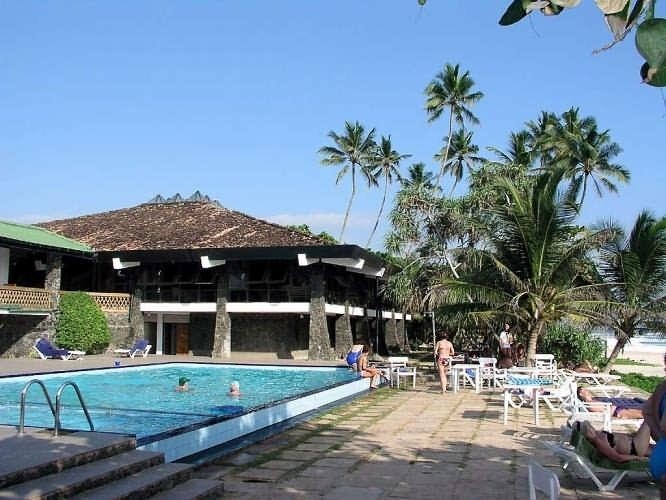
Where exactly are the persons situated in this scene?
[344,340,369,373]
[357,343,382,389]
[641,351,665,500]
[577,418,651,464]
[175,376,191,393]
[577,385,645,420]
[496,322,513,369]
[433,330,455,395]
[225,380,244,396]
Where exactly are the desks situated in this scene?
[112,348,131,358]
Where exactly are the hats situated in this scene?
[179,377,190,386]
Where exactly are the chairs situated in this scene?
[127,339,152,359]
[388,356,417,390]
[31,335,69,361]
[447,353,657,500]
